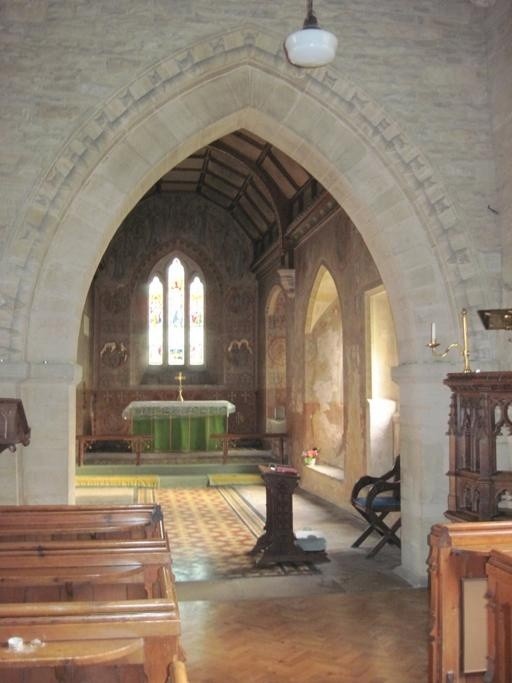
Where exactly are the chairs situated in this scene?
[351,454,400,558]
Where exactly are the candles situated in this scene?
[431,322,436,345]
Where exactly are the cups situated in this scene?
[8,637,23,654]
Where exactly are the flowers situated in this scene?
[303,450,320,465]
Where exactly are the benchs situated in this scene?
[77,435,153,466]
[1,503,188,683]
[210,432,288,466]
[425,519,512,682]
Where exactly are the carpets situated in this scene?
[73,474,160,488]
[131,486,322,583]
[207,472,265,486]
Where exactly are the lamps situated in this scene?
[284,0,337,68]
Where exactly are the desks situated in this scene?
[121,399,235,453]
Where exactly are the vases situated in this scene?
[308,458,315,465]
